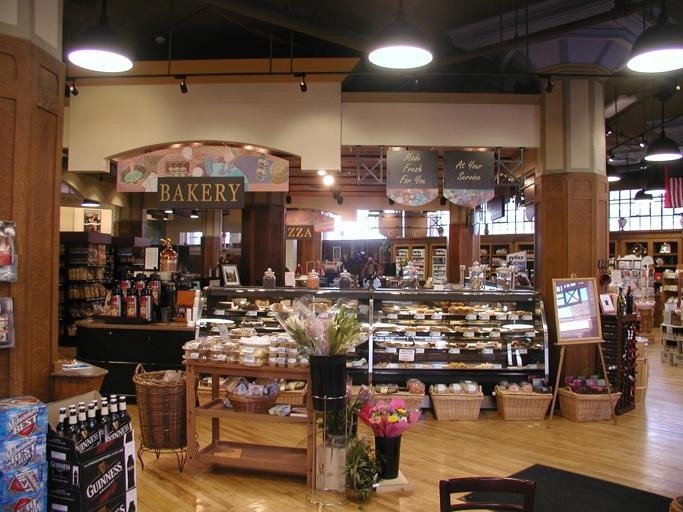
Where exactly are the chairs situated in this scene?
[438,476,539,511]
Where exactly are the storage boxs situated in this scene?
[1,386,140,512]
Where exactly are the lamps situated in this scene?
[621,2,683,74]
[367,0,436,72]
[63,0,135,76]
[644,86,683,165]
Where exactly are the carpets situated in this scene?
[460,461,675,512]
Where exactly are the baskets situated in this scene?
[496,389,553,419]
[634,339,648,404]
[230,398,277,414]
[197,385,226,405]
[431,394,483,420]
[558,387,622,422]
[374,394,424,409]
[275,387,308,406]
[132,363,187,448]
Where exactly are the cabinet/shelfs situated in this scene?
[58,231,151,345]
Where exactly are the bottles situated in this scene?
[139,289,151,319]
[124,288,137,317]
[338,270,351,289]
[109,289,121,317]
[120,274,131,296]
[262,267,275,288]
[135,274,145,294]
[307,270,318,288]
[57,395,130,445]
[149,274,159,304]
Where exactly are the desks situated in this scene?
[73,314,194,403]
